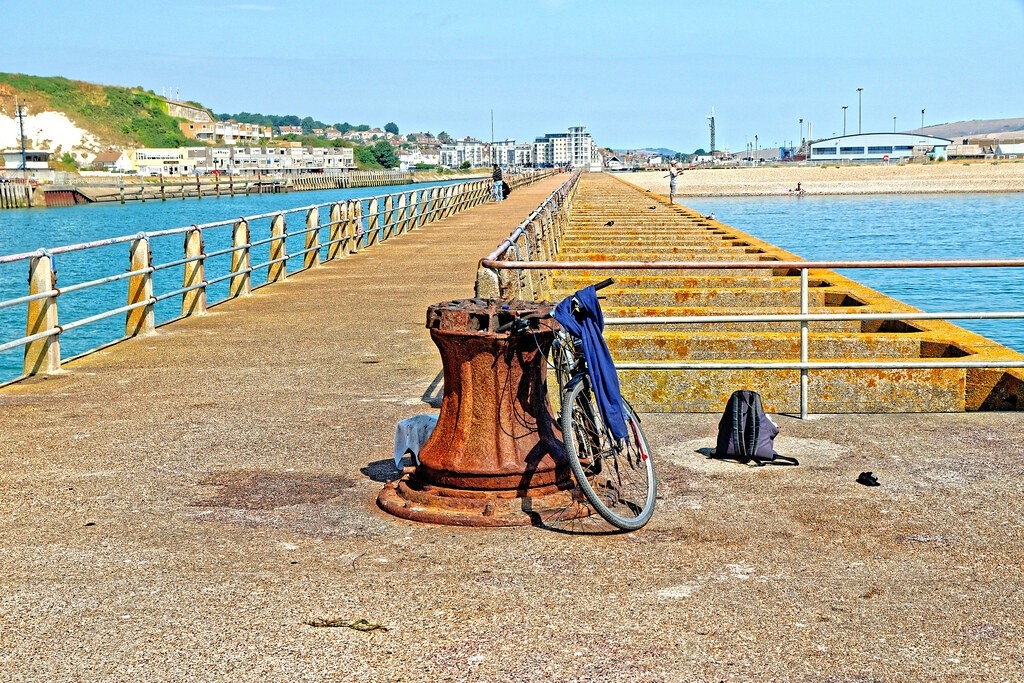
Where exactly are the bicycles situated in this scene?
[496,276,657,531]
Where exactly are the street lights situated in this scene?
[783,140,793,150]
[755,134,759,161]
[842,106,849,136]
[921,109,925,135]
[893,116,897,133]
[746,142,752,161]
[798,118,804,146]
[856,88,864,133]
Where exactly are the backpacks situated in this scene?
[710,389,779,463]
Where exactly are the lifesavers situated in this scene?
[559,167,562,173]
[884,155,889,161]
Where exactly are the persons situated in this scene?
[670,160,684,204]
[492,163,510,202]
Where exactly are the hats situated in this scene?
[670,160,678,165]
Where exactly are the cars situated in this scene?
[266,172,276,178]
[150,172,159,176]
[198,169,241,176]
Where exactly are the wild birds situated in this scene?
[649,206,656,209]
[646,188,651,192]
[700,212,714,220]
[604,221,614,226]
